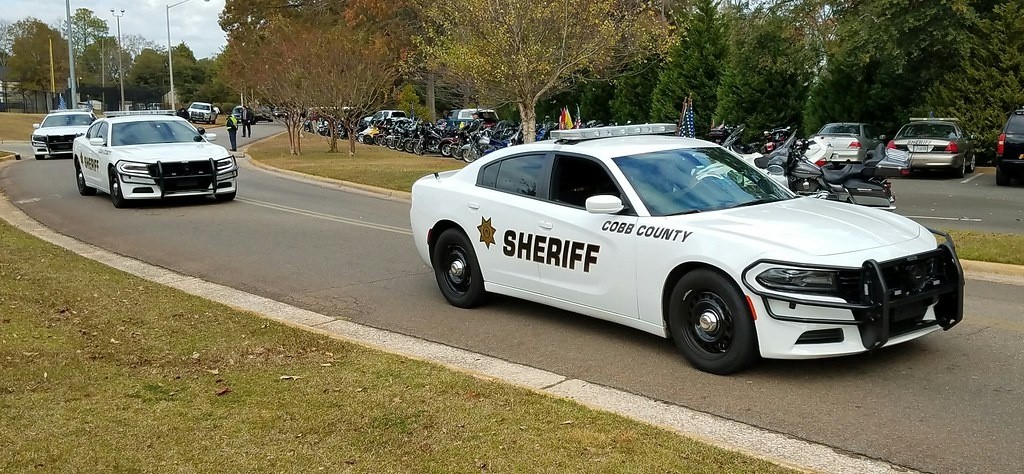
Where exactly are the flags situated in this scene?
[559,105,581,130]
[680,102,694,138]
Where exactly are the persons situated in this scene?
[213,106,220,115]
[226,112,238,151]
[240,106,251,138]
[178,104,189,119]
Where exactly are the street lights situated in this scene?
[166,0,209,112]
[110,8,126,111]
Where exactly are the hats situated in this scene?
[471,111,480,115]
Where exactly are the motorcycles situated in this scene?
[720,123,897,213]
[708,123,806,160]
[304,116,631,164]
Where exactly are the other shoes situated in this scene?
[241,134,251,138]
[230,149,236,151]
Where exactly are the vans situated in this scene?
[232,106,244,123]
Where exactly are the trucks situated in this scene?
[446,108,499,121]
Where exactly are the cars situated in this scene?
[254,106,273,122]
[409,123,967,373]
[134,104,147,110]
[187,102,216,124]
[73,111,239,209]
[29,109,99,159]
[885,116,974,176]
[272,106,288,117]
[803,121,885,165]
[147,103,162,110]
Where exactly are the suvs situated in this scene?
[363,110,407,122]
[994,108,1023,186]
